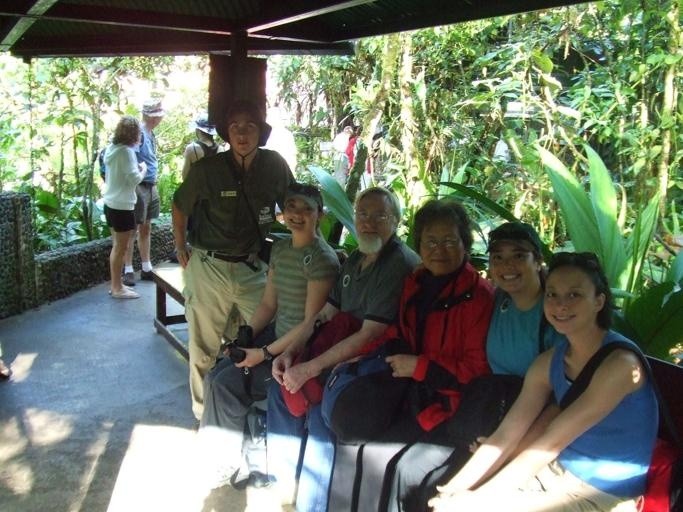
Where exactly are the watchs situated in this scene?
[262,345,273,360]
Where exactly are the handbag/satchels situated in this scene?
[281,312,356,418]
[320,336,415,443]
[448,374,523,447]
[638,436,681,511]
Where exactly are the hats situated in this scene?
[486,222,540,252]
[282,186,322,209]
[216,101,272,146]
[143,98,164,119]
[192,114,216,135]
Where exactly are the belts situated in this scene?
[206,251,259,271]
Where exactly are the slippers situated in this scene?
[107,285,138,298]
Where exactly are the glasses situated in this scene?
[548,250,600,268]
[422,235,460,248]
[288,183,322,205]
[487,229,539,251]
[356,210,392,223]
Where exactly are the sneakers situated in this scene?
[251,471,276,488]
[231,464,250,489]
[123,273,134,286]
[141,269,154,281]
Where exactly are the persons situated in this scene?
[331,126,353,184]
[259,185,422,510]
[102,116,148,300]
[345,127,372,191]
[122,97,167,286]
[190,184,340,454]
[170,99,296,422]
[384,222,566,511]
[426,250,660,511]
[325,200,497,510]
[179,114,225,180]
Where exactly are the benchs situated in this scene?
[145,260,189,362]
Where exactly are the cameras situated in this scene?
[229,325,253,364]
